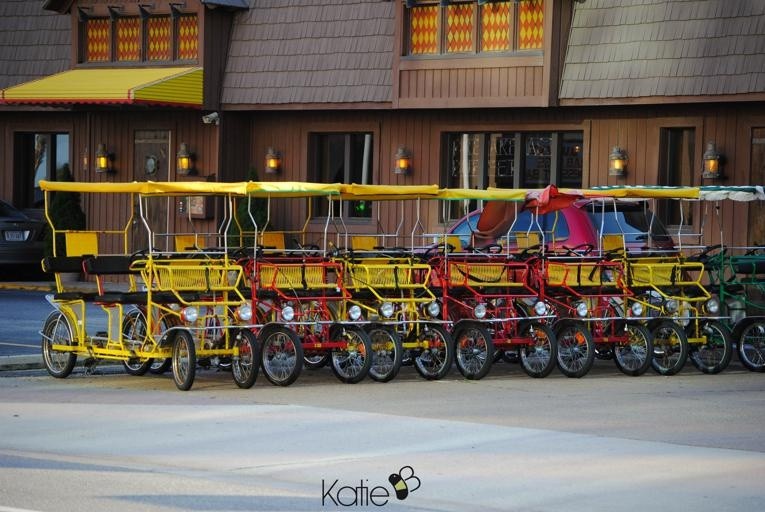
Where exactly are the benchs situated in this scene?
[735,260,765,282]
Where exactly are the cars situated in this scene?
[0,199,49,266]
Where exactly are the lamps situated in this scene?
[95,143,116,173]
[701,142,729,179]
[608,147,629,177]
[394,146,414,176]
[175,143,198,177]
[263,146,283,175]
